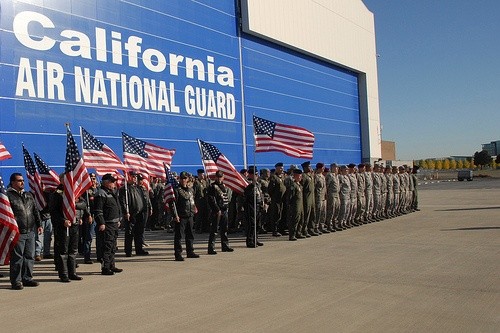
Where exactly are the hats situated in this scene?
[216,170,223,178]
[172,172,179,176]
[180,171,189,177]
[132,172,137,176]
[240,160,419,174]
[102,173,114,181]
[88,172,96,177]
[136,173,145,179]
[197,169,204,174]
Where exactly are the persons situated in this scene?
[54,173,93,283]
[208,170,233,255]
[119,171,152,257]
[150,160,421,240]
[6,172,43,290]
[84,172,123,275]
[35,205,54,261]
[173,171,199,261]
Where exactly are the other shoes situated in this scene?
[11,282,23,290]
[35,256,41,262]
[83,206,420,275]
[68,272,83,280]
[75,264,78,267]
[61,275,71,282]
[24,280,39,287]
[42,254,54,260]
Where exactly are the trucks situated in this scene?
[457,171,473,181]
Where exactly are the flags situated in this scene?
[122,133,176,179]
[162,164,180,211]
[0,140,20,266]
[200,139,249,193]
[82,127,130,173]
[61,127,92,224]
[252,114,314,159]
[22,146,47,211]
[95,167,155,194]
[34,153,62,190]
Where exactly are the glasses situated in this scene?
[90,178,96,181]
[15,179,24,182]
[180,177,188,179]
